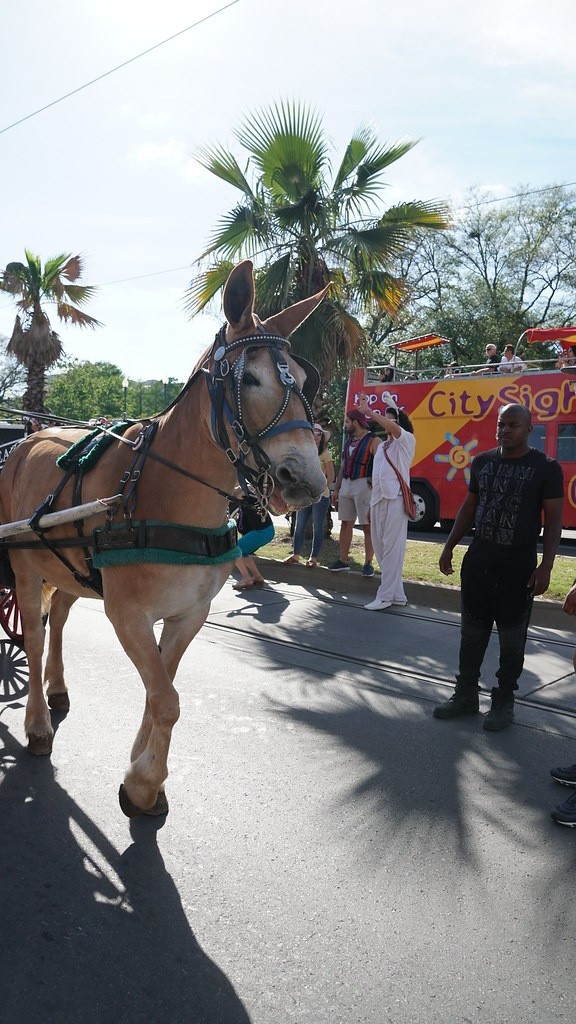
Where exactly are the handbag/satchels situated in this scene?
[400,479,416,520]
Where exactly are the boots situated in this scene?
[482,689,515,732]
[433,686,479,719]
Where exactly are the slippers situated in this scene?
[284,556,299,564]
[307,560,317,566]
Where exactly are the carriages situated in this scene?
[0,260,337,817]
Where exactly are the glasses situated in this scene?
[486,348,493,351]
[385,367,391,371]
[312,431,322,436]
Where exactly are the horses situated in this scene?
[1,258,336,818]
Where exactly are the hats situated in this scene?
[347,409,369,427]
[312,424,331,442]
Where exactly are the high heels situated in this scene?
[253,575,265,586]
[232,578,254,589]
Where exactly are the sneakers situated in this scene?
[550,792,576,827]
[550,764,576,786]
[364,599,392,610]
[361,564,374,577]
[328,559,350,571]
[392,598,408,605]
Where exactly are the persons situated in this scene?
[283,423,334,566]
[328,409,382,576]
[470,344,500,376]
[352,391,416,610]
[497,344,527,373]
[559,345,576,369]
[550,578,576,827]
[403,362,464,382]
[377,365,394,383]
[431,403,564,731]
[230,484,275,590]
